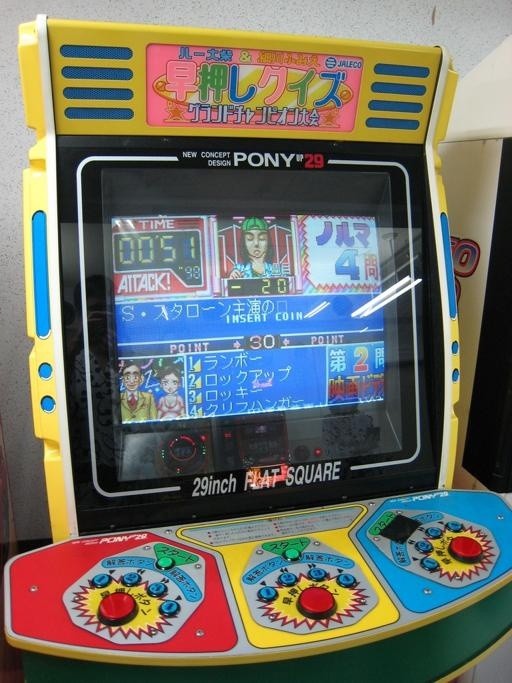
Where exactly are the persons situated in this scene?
[225,217,289,280]
[119,362,160,424]
[150,364,188,419]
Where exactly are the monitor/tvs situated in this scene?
[111,216,385,425]
